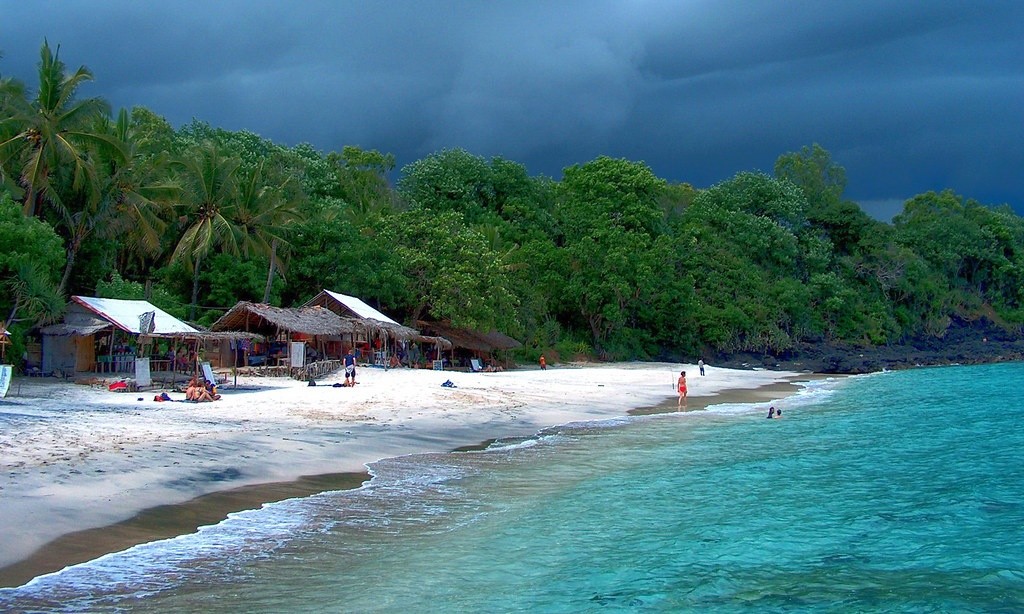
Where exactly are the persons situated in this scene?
[93,336,198,375]
[677,371,687,407]
[769,407,775,415]
[698,357,705,376]
[268,340,318,363]
[477,364,504,372]
[411,342,421,369]
[186,376,221,402]
[774,409,783,419]
[343,350,356,387]
[540,354,547,371]
[390,353,403,369]
